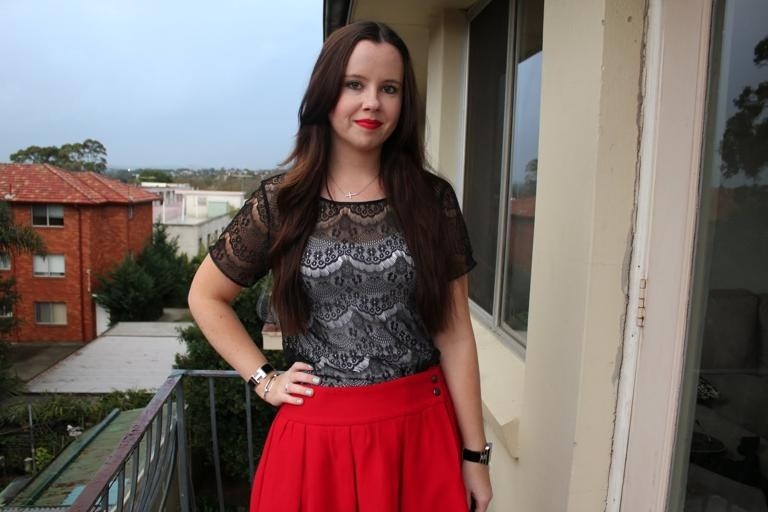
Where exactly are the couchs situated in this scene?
[693,288,768,488]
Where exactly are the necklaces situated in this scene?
[328,169,380,198]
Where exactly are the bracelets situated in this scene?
[462,443,491,466]
[246,362,273,390]
[263,373,278,400]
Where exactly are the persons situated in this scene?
[187,22,494,512]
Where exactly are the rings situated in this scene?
[283,382,289,392]
